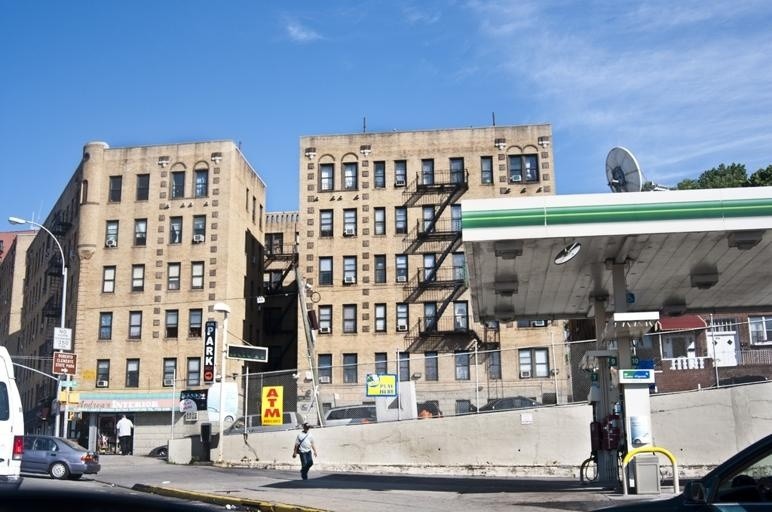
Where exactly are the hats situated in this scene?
[304,423,310,428]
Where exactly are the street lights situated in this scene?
[212,303,230,461]
[10,216,68,437]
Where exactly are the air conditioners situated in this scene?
[397,324,408,331]
[320,327,331,333]
[534,320,545,327]
[164,378,174,386]
[105,239,117,247]
[344,276,355,283]
[520,370,531,378]
[97,380,108,387]
[397,275,406,282]
[343,228,355,235]
[318,375,331,383]
[509,174,522,182]
[193,234,205,243]
[395,179,406,186]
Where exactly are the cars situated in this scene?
[594,434,772,512]
[479,395,543,412]
[21,435,101,480]
[149,435,216,460]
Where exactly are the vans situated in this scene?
[224,412,304,435]
[324,405,376,425]
[0,344,24,494]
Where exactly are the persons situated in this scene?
[293,422,318,481]
[115,414,133,455]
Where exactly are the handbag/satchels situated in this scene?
[297,445,301,454]
